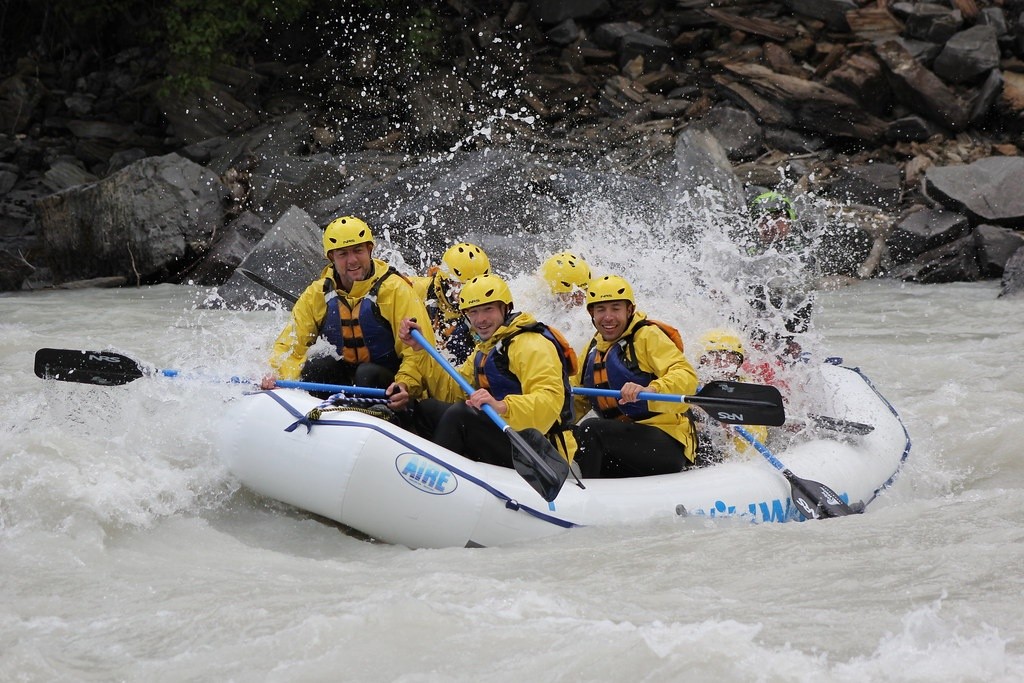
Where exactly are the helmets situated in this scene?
[439,243,491,284]
[542,252,591,296]
[323,216,375,258]
[749,192,796,224]
[696,328,744,363]
[458,274,514,311]
[586,276,635,317]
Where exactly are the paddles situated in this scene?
[696,386,857,520]
[806,411,876,437]
[406,315,570,504]
[231,266,300,305]
[572,377,786,428]
[33,346,402,414]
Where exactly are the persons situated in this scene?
[398,274,578,466]
[695,331,767,458]
[262,217,435,413]
[542,253,590,310]
[404,243,491,366]
[570,276,699,479]
[738,192,812,356]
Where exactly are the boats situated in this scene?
[220,331,910,546]
